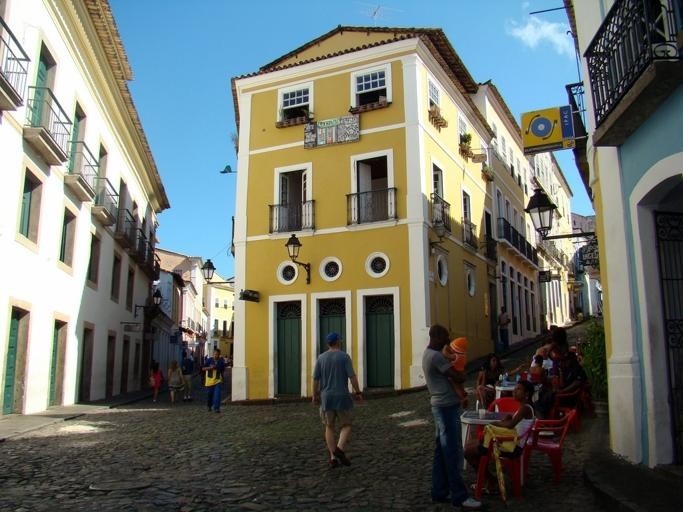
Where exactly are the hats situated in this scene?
[326,332,342,342]
[450,337,468,353]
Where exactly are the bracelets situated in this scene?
[357,392,361,394]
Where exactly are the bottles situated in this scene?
[503,375,507,386]
[499,374,503,386]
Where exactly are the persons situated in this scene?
[498,306,513,356]
[202,349,226,413]
[476,354,505,409]
[464,379,534,497]
[149,362,165,403]
[166,360,186,403]
[527,327,587,417]
[181,351,194,402]
[443,337,469,409]
[422,324,482,510]
[311,333,363,468]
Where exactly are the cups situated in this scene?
[479,409,485,420]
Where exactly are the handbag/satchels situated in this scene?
[148,370,155,386]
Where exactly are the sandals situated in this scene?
[470,480,486,490]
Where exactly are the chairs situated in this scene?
[476,397,522,441]
[581,393,594,419]
[467,417,540,504]
[525,410,578,486]
[521,372,548,386]
[548,390,581,436]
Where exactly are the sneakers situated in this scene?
[329,459,338,467]
[207,406,212,412]
[215,409,220,413]
[333,447,351,466]
[462,498,480,507]
